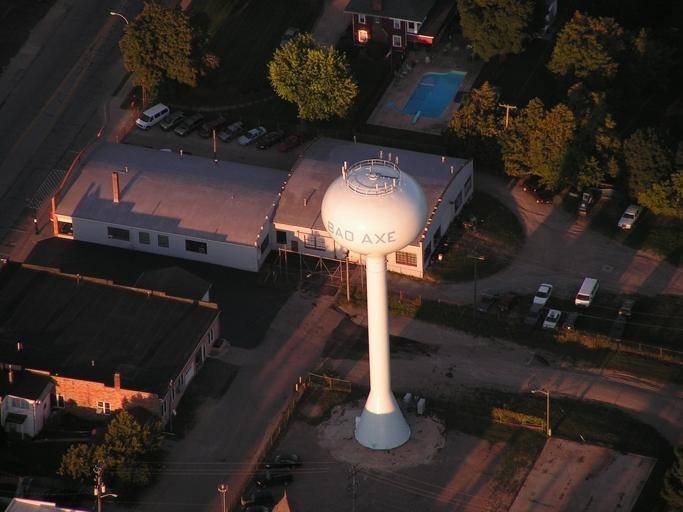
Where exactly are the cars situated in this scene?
[156,110,188,130]
[480,282,579,333]
[238,452,302,512]
[198,112,228,139]
[617,297,636,318]
[216,119,303,153]
[616,204,644,231]
[173,112,204,136]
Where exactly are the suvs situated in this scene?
[134,101,171,131]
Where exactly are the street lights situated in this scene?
[97,493,120,512]
[109,11,129,25]
[471,256,485,321]
[23,198,38,234]
[530,389,550,438]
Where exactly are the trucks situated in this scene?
[574,277,601,308]
